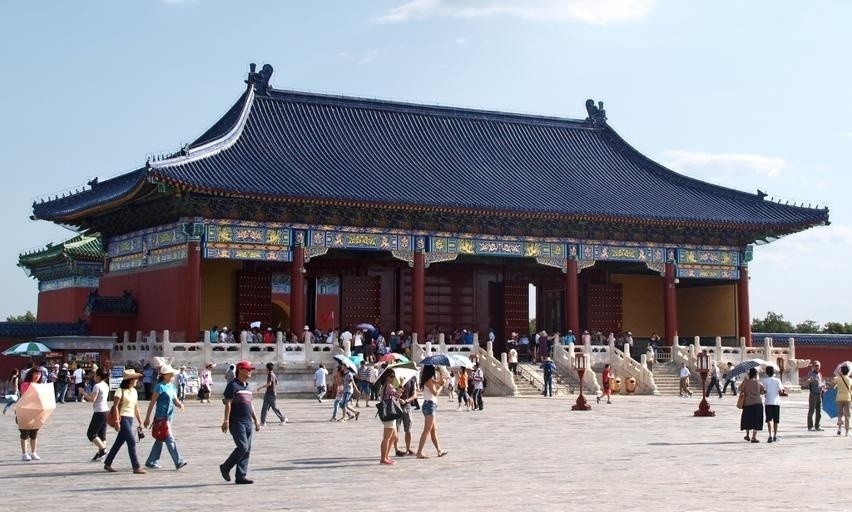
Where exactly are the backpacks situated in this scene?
[478,369,487,387]
[57,370,69,383]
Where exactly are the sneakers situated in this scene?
[153,463,161,469]
[22,454,31,461]
[329,412,359,422]
[177,460,187,470]
[98,448,108,457]
[815,428,824,431]
[380,449,447,464]
[808,428,815,431]
[32,453,40,460]
[767,437,772,442]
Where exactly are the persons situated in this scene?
[301,325,340,350]
[390,330,411,352]
[2,360,235,415]
[451,328,473,351]
[565,330,576,345]
[597,364,615,404]
[220,360,260,484]
[593,331,606,345]
[806,359,825,431]
[14,366,44,460]
[825,362,852,436]
[450,361,484,411]
[763,366,787,442]
[506,330,556,397]
[488,328,495,342]
[77,367,109,463]
[256,361,289,425]
[737,364,764,442]
[329,360,371,422]
[143,363,188,472]
[678,358,738,399]
[104,367,146,475]
[392,377,417,456]
[340,327,386,361]
[616,331,660,364]
[313,362,329,403]
[376,368,403,466]
[414,366,449,458]
[210,323,298,351]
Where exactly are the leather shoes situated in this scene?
[104,465,116,472]
[744,436,750,441]
[751,438,759,443]
[235,478,253,484]
[220,464,231,481]
[134,469,146,474]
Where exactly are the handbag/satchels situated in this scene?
[151,417,169,441]
[81,382,87,387]
[198,386,210,399]
[604,382,609,388]
[376,383,403,421]
[737,382,745,409]
[107,388,123,427]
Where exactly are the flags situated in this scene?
[321,310,335,323]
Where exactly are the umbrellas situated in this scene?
[721,359,761,379]
[0,341,52,368]
[834,360,852,378]
[12,381,58,432]
[822,386,844,419]
[744,357,781,377]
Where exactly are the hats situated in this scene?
[304,326,309,330]
[236,361,256,369]
[813,361,820,366]
[595,332,602,335]
[160,365,180,374]
[544,357,552,361]
[540,331,547,337]
[123,369,142,380]
[568,330,572,333]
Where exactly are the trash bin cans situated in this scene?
[610,377,621,395]
[625,377,636,395]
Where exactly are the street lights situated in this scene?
[468,353,479,372]
[571,351,592,410]
[693,348,716,417]
[776,355,788,397]
